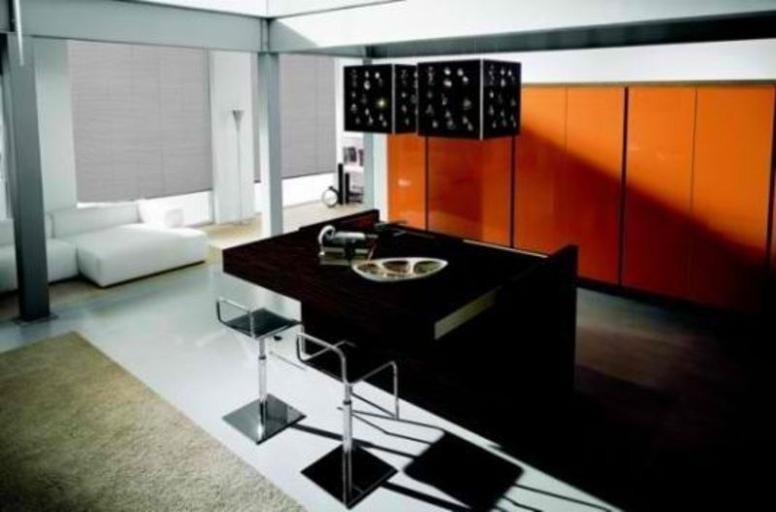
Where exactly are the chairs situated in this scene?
[0,213,78,293]
[297,333,397,507]
[51,202,207,294]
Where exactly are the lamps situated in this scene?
[231,109,250,225]
[418,59,521,138]
[344,64,418,133]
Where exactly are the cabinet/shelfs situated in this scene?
[513,83,626,286]
[386,132,512,246]
[621,81,775,312]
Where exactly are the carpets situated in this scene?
[0,331,305,512]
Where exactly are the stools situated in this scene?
[216,297,303,444]
